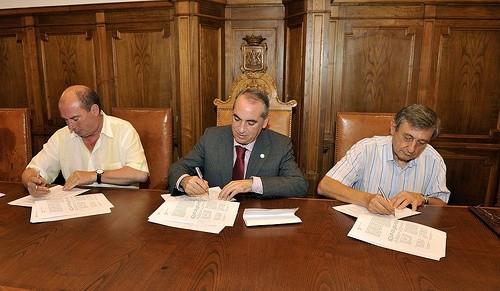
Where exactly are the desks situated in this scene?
[0,180,500,291]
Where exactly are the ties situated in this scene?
[232,145,247,181]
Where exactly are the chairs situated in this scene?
[110,106,172,190]
[334,111,397,166]
[0,107,33,182]
[214,72,297,137]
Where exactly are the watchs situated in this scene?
[96,169,104,184]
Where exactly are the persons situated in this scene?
[168,88,309,201]
[317,104,451,215]
[21,85,150,198]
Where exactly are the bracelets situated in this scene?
[421,194,429,204]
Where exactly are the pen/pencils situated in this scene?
[36,169,40,190]
[379,186,396,216]
[195,166,209,195]
